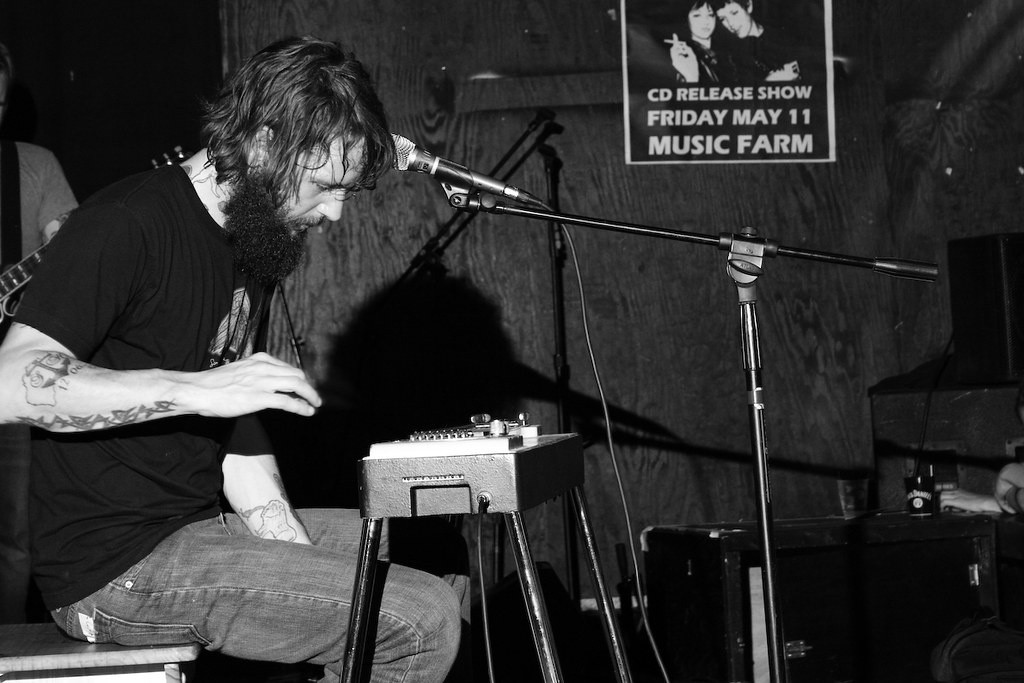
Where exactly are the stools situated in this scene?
[0,623,200,683]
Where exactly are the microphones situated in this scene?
[391,133,543,207]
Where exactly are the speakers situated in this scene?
[641,508,1000,683]
[947,230,1024,388]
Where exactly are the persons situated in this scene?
[0,44,79,620]
[0,34,471,683]
[938,384,1024,517]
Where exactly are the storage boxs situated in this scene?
[644,505,995,683]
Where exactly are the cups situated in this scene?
[837,479,868,519]
[904,475,934,519]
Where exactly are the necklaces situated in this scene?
[218,262,249,366]
